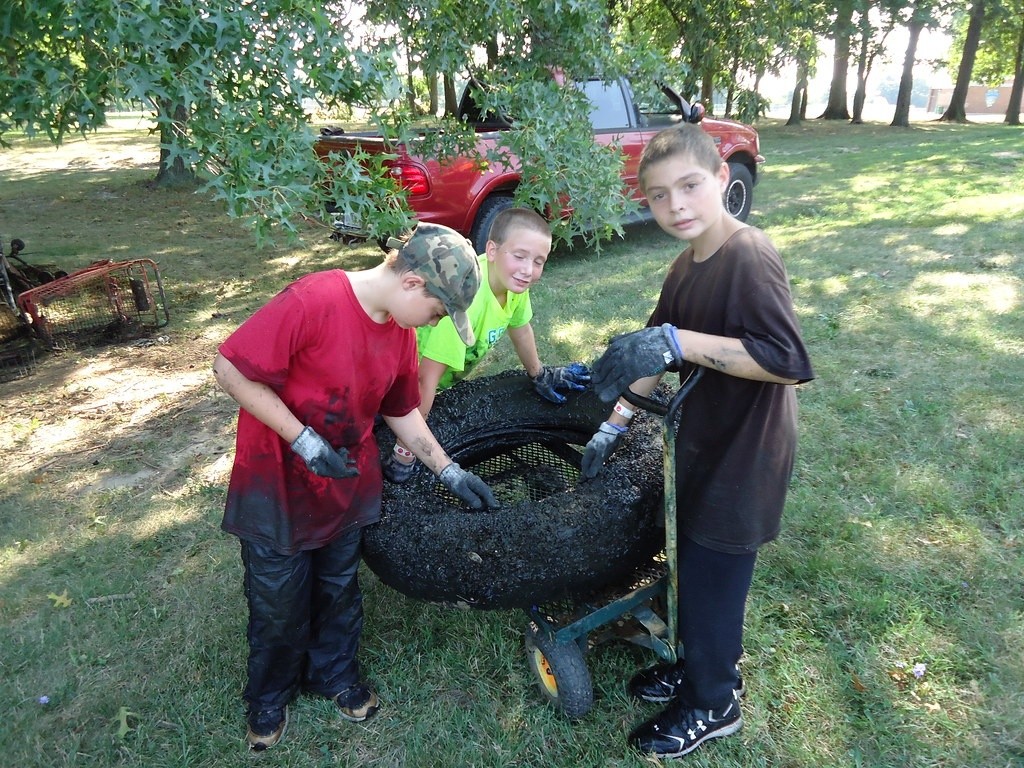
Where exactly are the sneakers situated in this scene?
[627,688,743,758]
[630,661,746,702]
[244,703,290,751]
[331,679,383,723]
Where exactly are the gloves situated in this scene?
[384,453,416,484]
[438,462,501,510]
[526,363,590,404]
[590,322,683,404]
[290,425,361,479]
[581,421,629,480]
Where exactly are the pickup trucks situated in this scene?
[310,61,764,267]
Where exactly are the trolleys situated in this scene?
[0,238,169,384]
[397,360,705,716]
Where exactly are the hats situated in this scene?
[387,220,482,348]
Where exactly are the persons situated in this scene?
[211,217,503,751]
[582,122,816,761]
[383,208,594,481]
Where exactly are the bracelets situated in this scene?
[614,402,636,419]
[394,443,418,458]
